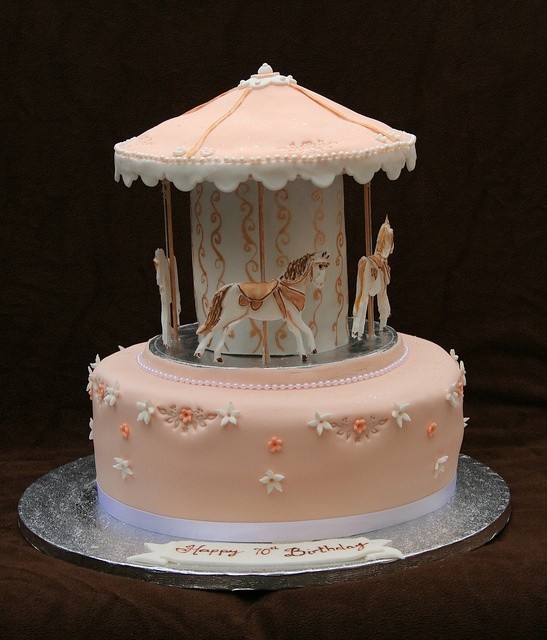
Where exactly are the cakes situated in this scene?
[86,62,470,541]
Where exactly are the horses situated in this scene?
[351,216,396,341]
[194,253,331,365]
[153,247,181,346]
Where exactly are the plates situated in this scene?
[16,444,512,591]
